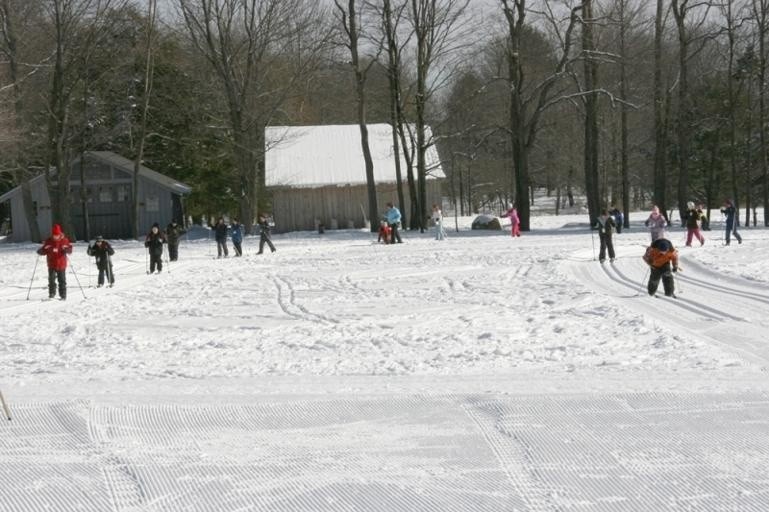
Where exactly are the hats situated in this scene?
[152,223,160,228]
[52,224,61,236]
[658,241,670,251]
[601,210,608,215]
[651,205,659,213]
[96,236,104,242]
[687,202,695,208]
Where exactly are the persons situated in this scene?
[144,222,166,274]
[378,202,443,245]
[166,218,187,262]
[255,214,277,255]
[609,207,623,233]
[644,199,743,248]
[592,207,616,264]
[641,238,680,297]
[37,223,72,301]
[86,233,115,287]
[229,217,243,258]
[500,203,521,237]
[208,216,228,257]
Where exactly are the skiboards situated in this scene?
[88,284,114,288]
[650,290,676,299]
[146,268,163,275]
[41,298,66,301]
[599,257,617,264]
